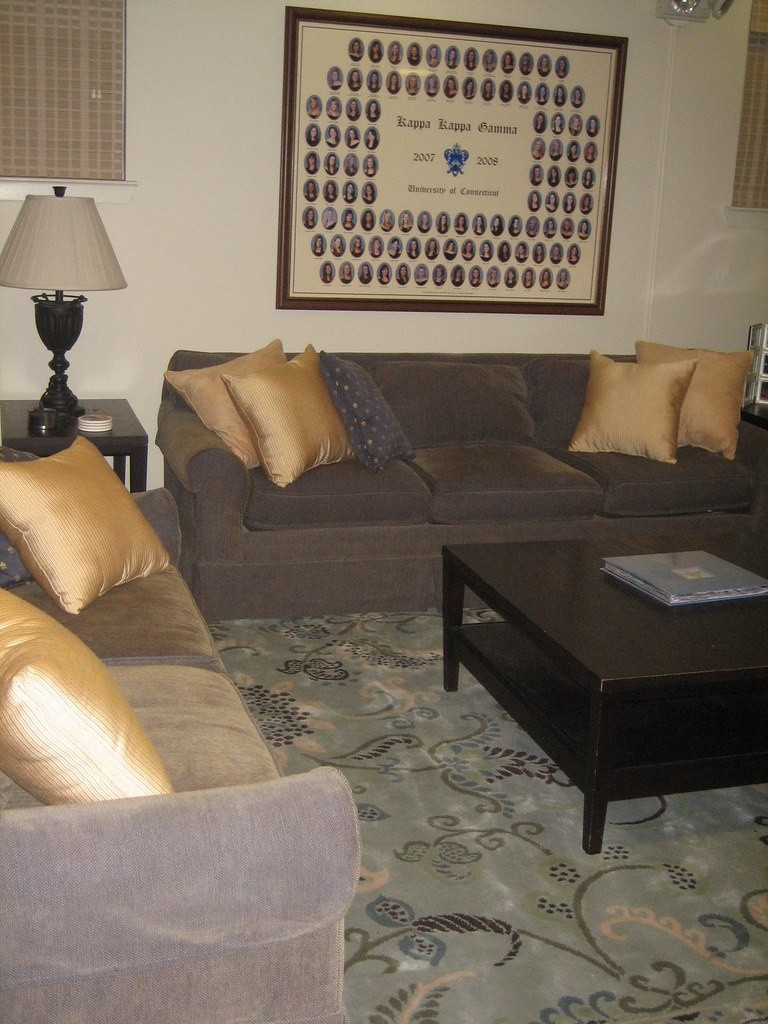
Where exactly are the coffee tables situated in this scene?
[442,532,768,854]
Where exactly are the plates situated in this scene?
[78,415,112,432]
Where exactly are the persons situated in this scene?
[304,37,598,289]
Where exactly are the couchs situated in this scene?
[155,349,768,624]
[0,487,361,1024]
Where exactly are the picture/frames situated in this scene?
[275,5,629,316]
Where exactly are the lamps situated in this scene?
[654,0,734,24]
[0,185,128,419]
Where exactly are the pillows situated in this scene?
[0,446,36,590]
[527,356,636,449]
[371,361,541,449]
[163,338,288,469]
[219,344,356,488]
[0,434,176,614]
[634,340,756,461]
[566,349,700,464]
[1,588,174,805]
[319,350,416,473]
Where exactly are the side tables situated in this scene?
[0,400,149,493]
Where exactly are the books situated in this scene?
[598,551,768,608]
